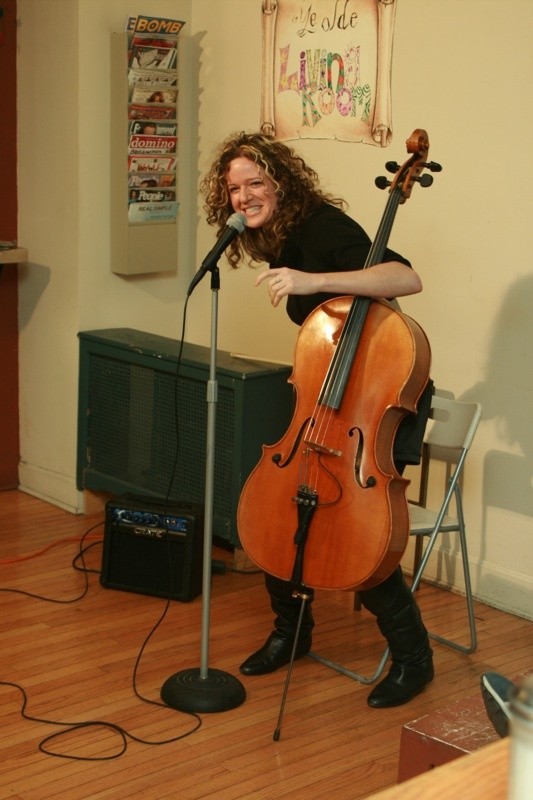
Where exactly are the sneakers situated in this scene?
[481,672,519,739]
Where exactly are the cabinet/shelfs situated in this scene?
[111,29,179,277]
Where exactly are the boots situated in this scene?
[367,600,435,708]
[240,571,315,676]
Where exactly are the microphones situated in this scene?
[186,211,248,296]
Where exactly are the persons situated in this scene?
[201,128,435,708]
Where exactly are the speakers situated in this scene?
[75,328,297,550]
[99,492,205,604]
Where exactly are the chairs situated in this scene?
[309,392,481,684]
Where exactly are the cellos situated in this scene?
[235,128,443,743]
